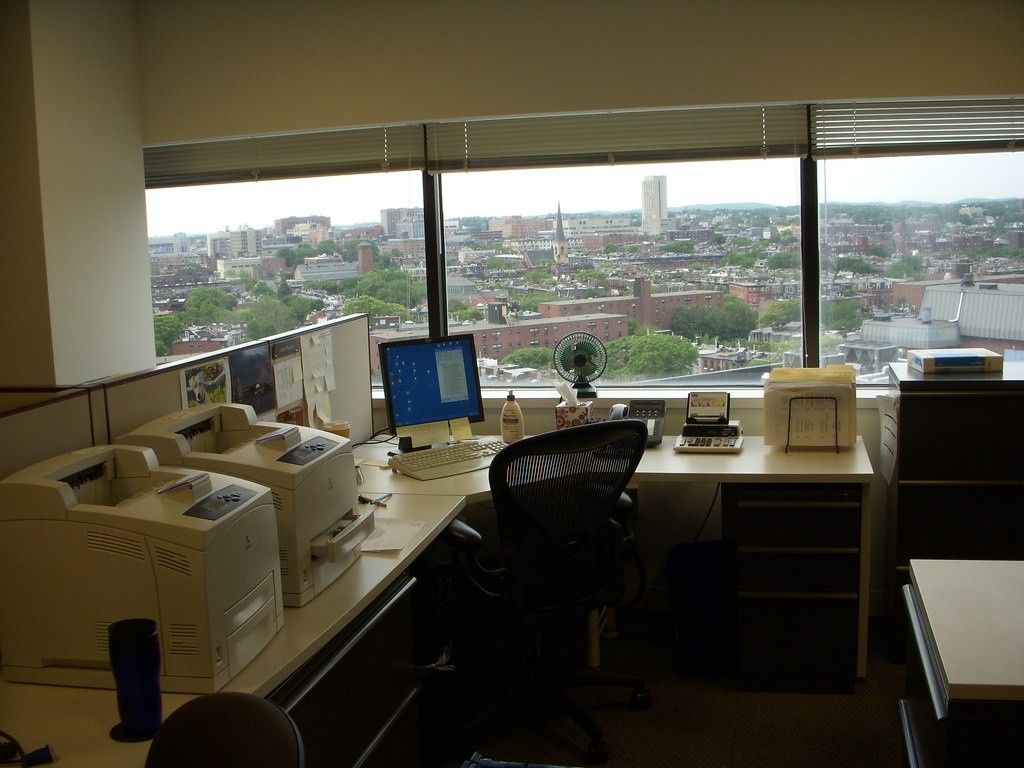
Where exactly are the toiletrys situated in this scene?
[500,390,524,444]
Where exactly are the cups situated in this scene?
[108,617,163,737]
[322,420,351,438]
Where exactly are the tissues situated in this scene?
[553,381,594,430]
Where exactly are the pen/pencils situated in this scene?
[370,492,392,504]
[359,495,387,507]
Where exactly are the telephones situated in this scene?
[607,399,666,447]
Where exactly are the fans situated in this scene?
[553,331,608,398]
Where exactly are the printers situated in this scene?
[0,443,284,696]
[114,401,376,608]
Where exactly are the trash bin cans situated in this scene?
[671,540,738,679]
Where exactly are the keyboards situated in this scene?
[389,439,509,481]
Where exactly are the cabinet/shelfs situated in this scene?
[720,483,861,694]
[877,376,1024,684]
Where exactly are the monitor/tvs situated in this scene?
[377,333,484,456]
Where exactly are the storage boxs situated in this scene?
[555,401,595,431]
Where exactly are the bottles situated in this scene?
[500,390,524,443]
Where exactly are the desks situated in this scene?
[352,434,874,680]
[0,496,467,768]
[899,559,1024,768]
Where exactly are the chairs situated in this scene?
[143,692,307,768]
[412,421,649,764]
